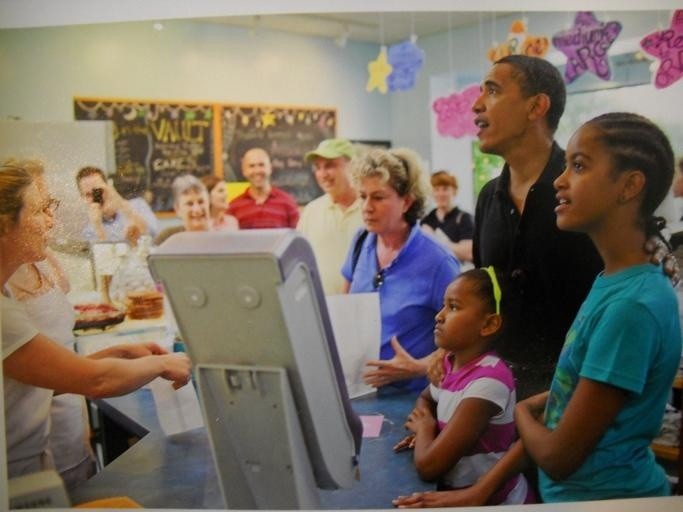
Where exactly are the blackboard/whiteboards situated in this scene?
[74,96,336,219]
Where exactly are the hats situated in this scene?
[301,135,357,163]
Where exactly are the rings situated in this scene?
[655,239,666,249]
[667,252,677,264]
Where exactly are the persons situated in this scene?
[391,112,683,509]
[472,54,681,506]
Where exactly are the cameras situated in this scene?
[89,187,104,203]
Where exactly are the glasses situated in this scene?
[39,194,62,212]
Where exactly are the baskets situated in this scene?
[127,290,167,319]
